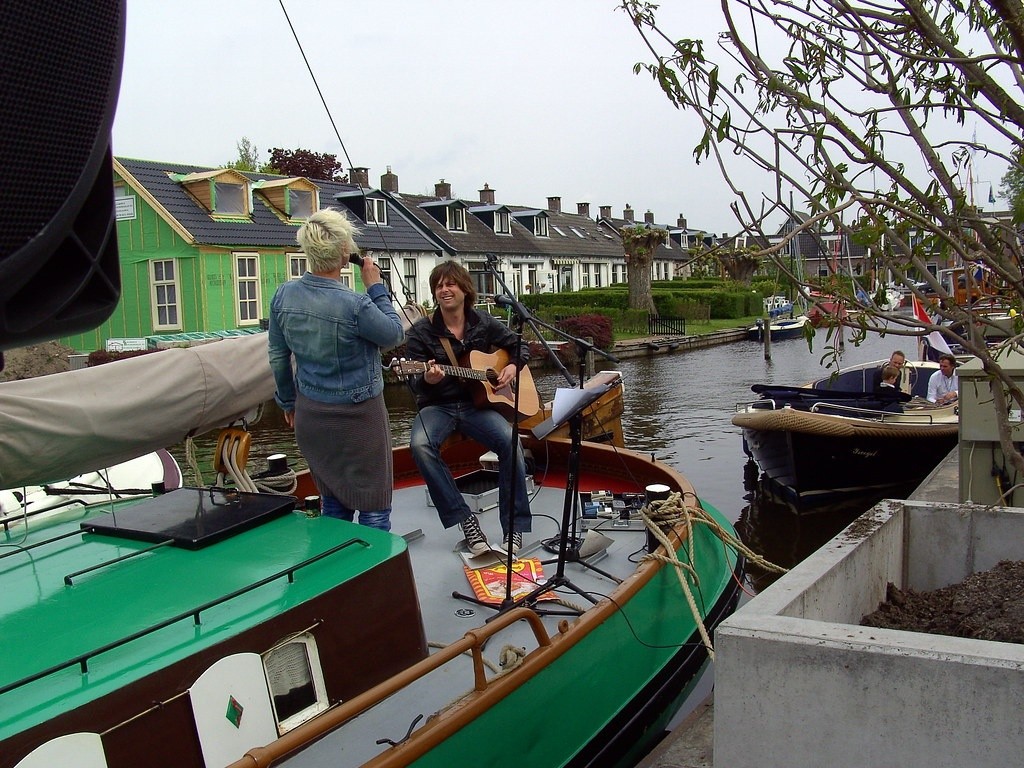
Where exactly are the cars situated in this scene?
[885,278,927,295]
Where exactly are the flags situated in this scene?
[973,260,983,279]
[912,290,955,356]
[988,186,996,202]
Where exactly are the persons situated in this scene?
[926,354,958,405]
[405,261,532,554]
[267,207,404,533]
[873,351,914,401]
[803,280,810,309]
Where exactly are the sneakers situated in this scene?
[458,513,488,554]
[500,533,522,554]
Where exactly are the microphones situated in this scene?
[349,253,388,279]
[493,295,513,305]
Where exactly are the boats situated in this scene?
[732,356,961,504]
[1,252,747,768]
[855,288,1022,365]
[746,314,815,343]
[808,292,849,328]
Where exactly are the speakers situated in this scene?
[0,0,127,352]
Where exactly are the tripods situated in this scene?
[453,253,624,652]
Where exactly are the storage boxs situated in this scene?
[424,468,534,515]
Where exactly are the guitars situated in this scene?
[383,348,539,422]
